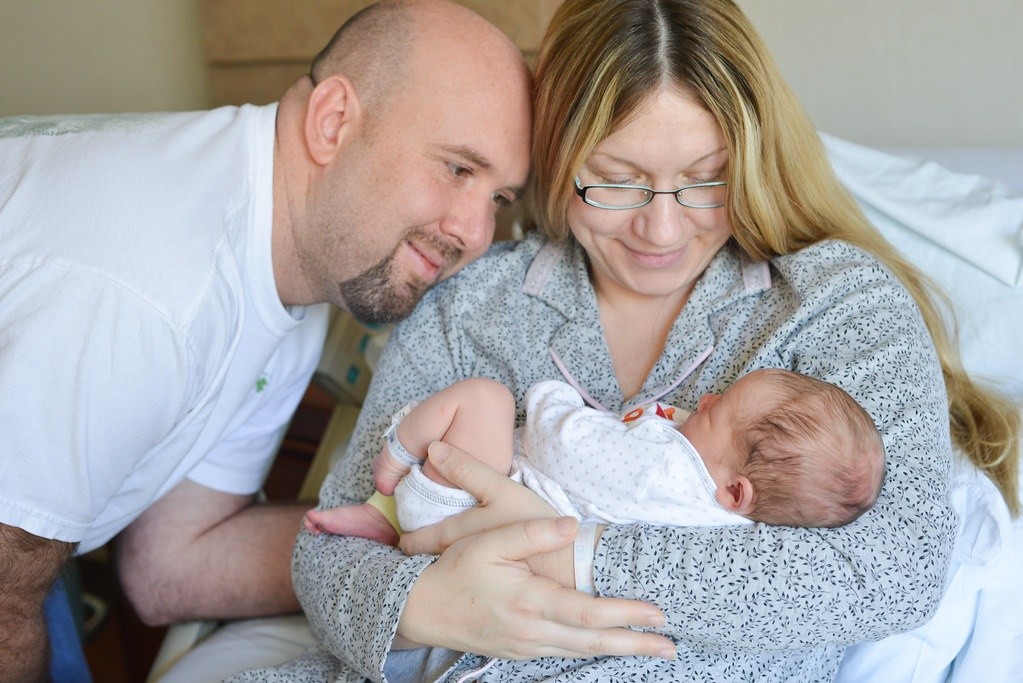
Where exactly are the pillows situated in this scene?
[818,133,1023,406]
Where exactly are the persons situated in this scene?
[221,0,1023,683]
[0,0,533,683]
[303,367,886,549]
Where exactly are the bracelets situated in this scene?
[574,521,599,594]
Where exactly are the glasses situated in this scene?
[574,175,727,210]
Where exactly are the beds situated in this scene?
[145,140,1023,683]
